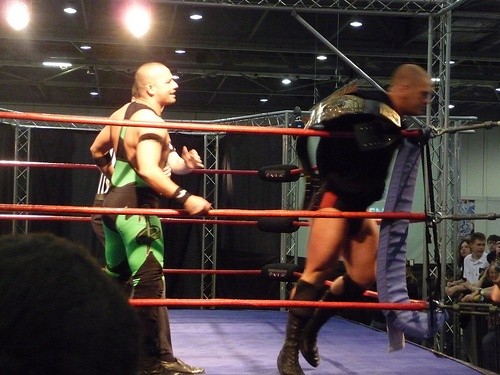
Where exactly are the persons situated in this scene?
[1,232,147,375]
[444,232,500,373]
[277,64,433,375]
[90,62,212,375]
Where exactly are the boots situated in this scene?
[277,312,313,375]
[300,300,334,367]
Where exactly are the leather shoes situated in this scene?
[156,357,205,375]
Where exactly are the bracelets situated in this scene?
[172,186,190,205]
[478,288,482,294]
[93,152,112,167]
